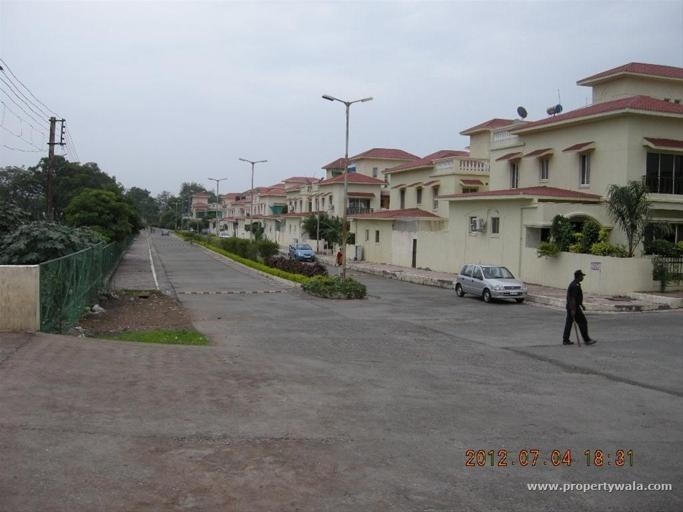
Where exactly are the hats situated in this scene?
[574,270,585,277]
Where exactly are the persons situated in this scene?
[563,270,597,345]
[336,249,342,272]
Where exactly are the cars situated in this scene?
[288,243,315,263]
[162,230,168,236]
[218,230,230,238]
[455,264,527,302]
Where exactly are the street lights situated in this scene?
[321,95,372,281]
[208,178,226,236]
[238,159,266,245]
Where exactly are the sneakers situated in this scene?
[563,340,574,345]
[586,339,596,345]
